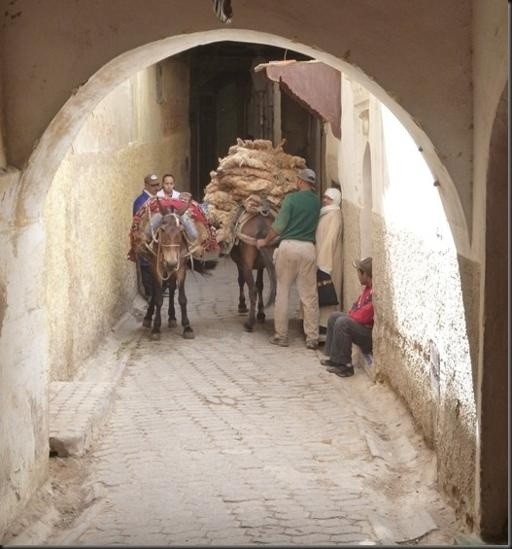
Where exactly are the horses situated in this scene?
[140,196,220,340]
[229,210,280,333]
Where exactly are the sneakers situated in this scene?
[267,334,289,347]
[306,340,319,350]
[320,359,354,377]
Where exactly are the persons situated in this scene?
[319,256,374,378]
[156,174,181,200]
[255,168,321,350]
[132,173,161,303]
[297,187,344,333]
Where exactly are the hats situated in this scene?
[295,168,317,184]
[352,256,372,278]
[144,174,160,185]
